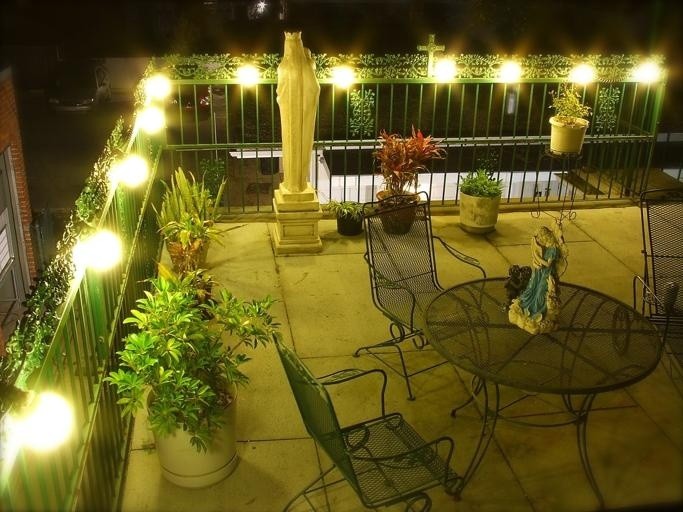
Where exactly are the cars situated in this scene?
[50,63,112,111]
[165,86,229,112]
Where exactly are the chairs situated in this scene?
[337,186,490,402]
[628,179,682,388]
[257,325,465,511]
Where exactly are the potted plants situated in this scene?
[545,83,595,156]
[94,272,280,491]
[455,163,504,238]
[149,164,249,278]
[317,125,449,239]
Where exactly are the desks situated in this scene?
[422,274,662,512]
